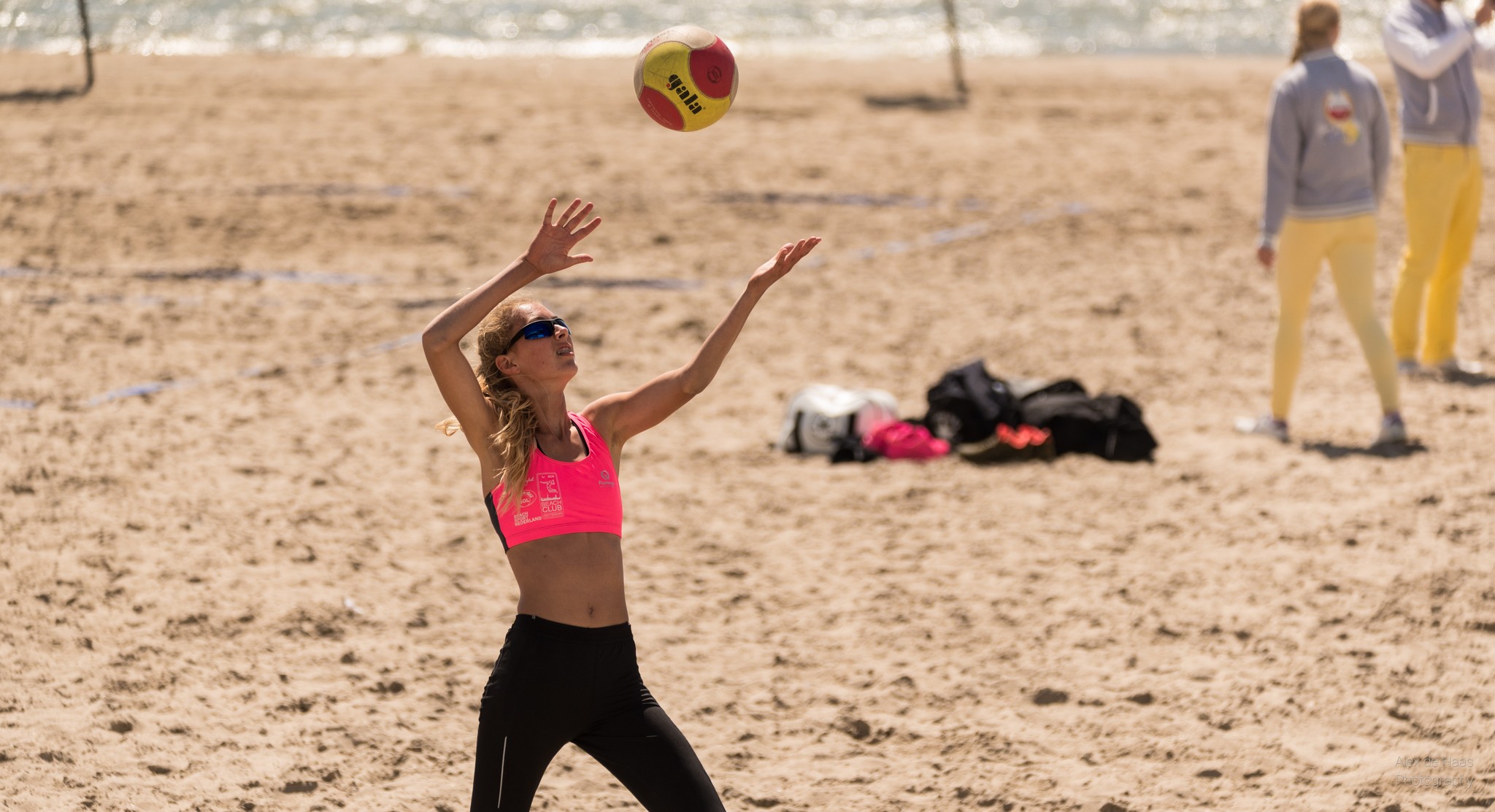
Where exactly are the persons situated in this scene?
[1234,0,1407,450]
[420,197,822,812]
[1377,0,1495,387]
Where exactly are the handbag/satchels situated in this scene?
[776,382,901,459]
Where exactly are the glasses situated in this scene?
[496,318,571,379]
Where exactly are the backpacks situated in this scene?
[1020,377,1158,467]
[926,357,1024,449]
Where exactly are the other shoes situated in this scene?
[1441,356,1492,382]
[1233,413,1291,443]
[1398,356,1443,380]
[1371,411,1408,448]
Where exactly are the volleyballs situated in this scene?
[634,25,739,132]
